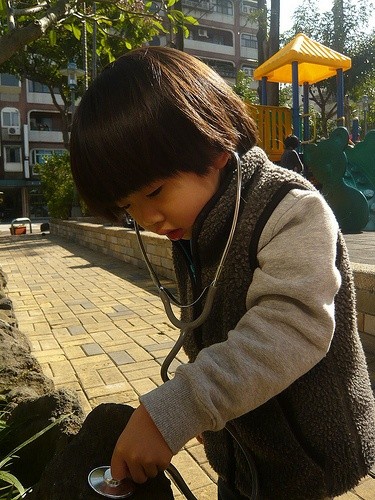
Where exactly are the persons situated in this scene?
[69,46,375,500]
[280,134,303,173]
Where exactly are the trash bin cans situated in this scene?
[9,225,27,235]
